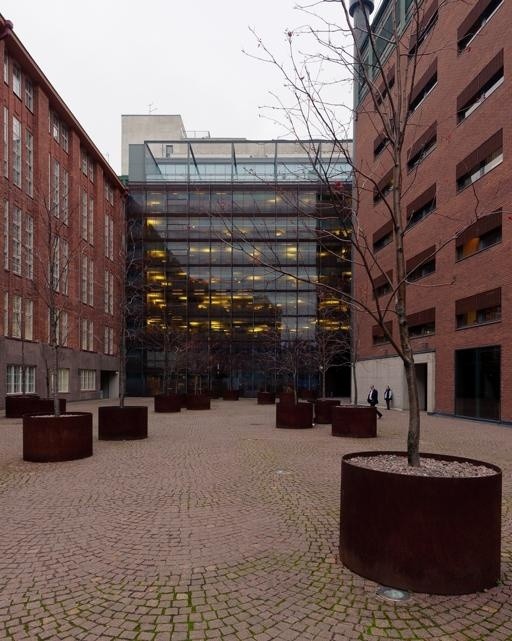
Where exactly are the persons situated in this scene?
[384,385,392,410]
[368,384,382,419]
